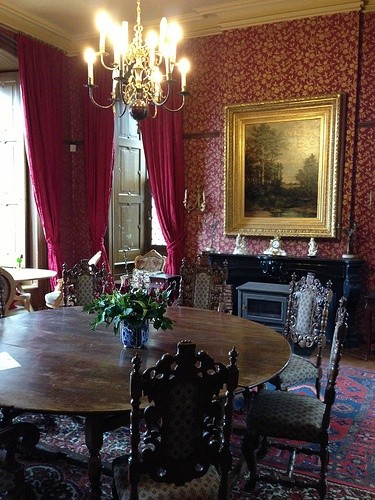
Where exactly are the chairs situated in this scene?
[112,339,240,500]
[62,259,107,308]
[134,249,164,272]
[241,294,350,500]
[257,272,333,398]
[0,268,31,316]
[173,250,233,315]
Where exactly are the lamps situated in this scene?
[83,0,191,122]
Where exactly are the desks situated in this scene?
[0,306,292,500]
[363,292,375,361]
[3,267,57,281]
[201,252,365,349]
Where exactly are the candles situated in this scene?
[202,191,206,204]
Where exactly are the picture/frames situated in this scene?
[223,93,347,241]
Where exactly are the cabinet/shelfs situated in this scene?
[235,281,291,336]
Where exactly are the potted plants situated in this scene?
[81,290,176,348]
[185,189,188,200]
